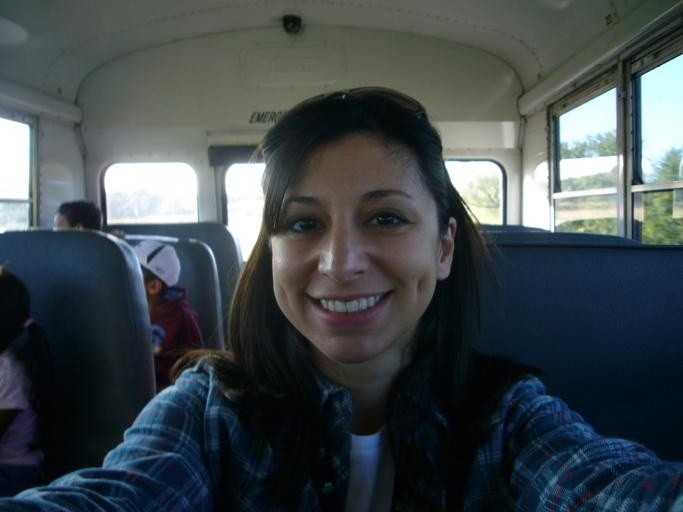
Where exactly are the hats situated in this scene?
[133,240,182,286]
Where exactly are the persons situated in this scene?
[0,268,56,498]
[1,84,683,512]
[53,199,100,230]
[132,239,203,394]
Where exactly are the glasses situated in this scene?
[289,86,423,119]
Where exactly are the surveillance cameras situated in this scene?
[283,15,301,34]
